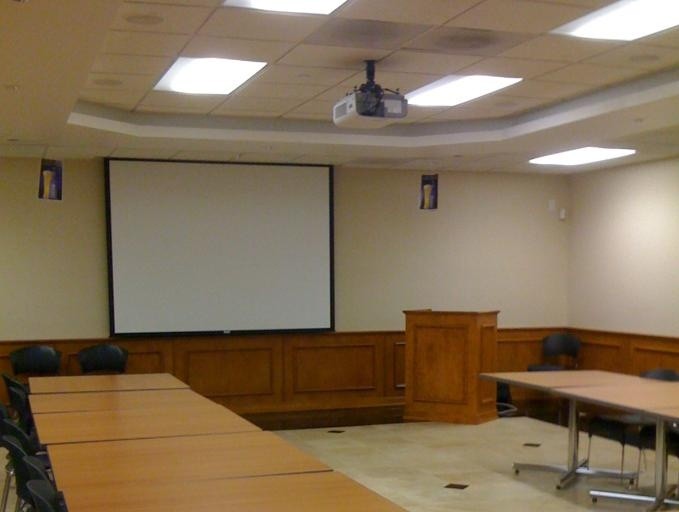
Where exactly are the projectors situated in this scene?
[332,91,408,130]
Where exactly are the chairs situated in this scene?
[7,339,129,375]
[0,373,59,512]
[527,333,579,371]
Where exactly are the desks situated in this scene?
[478,369,679,512]
